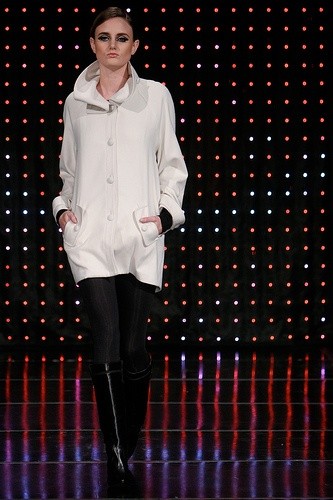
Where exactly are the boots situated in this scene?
[121,376,150,464]
[90,362,143,498]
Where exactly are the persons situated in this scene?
[52,6,189,500]
